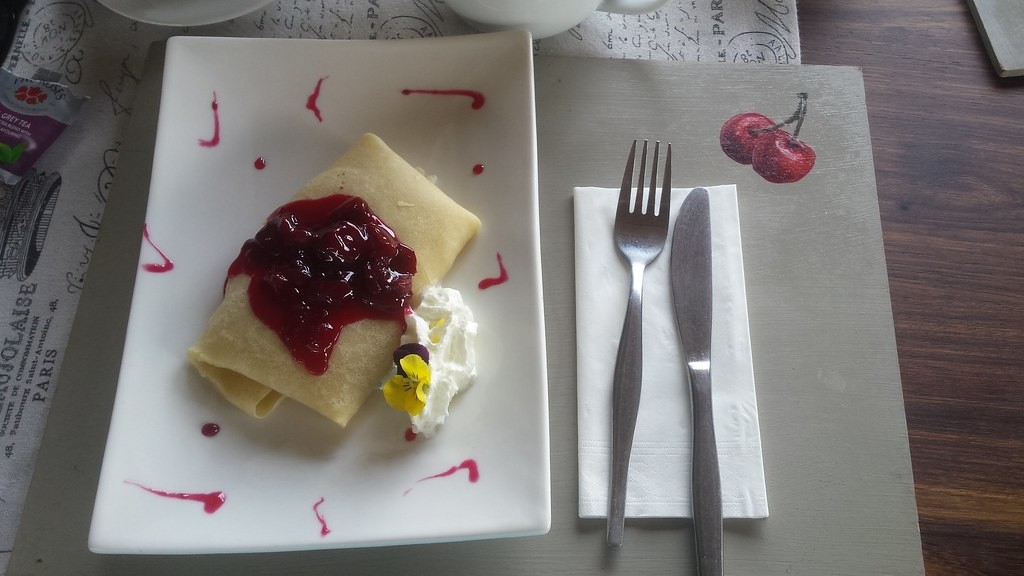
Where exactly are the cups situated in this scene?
[444,0,668,40]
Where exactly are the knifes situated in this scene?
[670,187,724,576]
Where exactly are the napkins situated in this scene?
[574,185,769,519]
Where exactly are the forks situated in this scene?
[606,139,671,549]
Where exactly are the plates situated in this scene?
[86,30,553,555]
[95,0,275,27]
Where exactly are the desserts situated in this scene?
[180,133,482,427]
